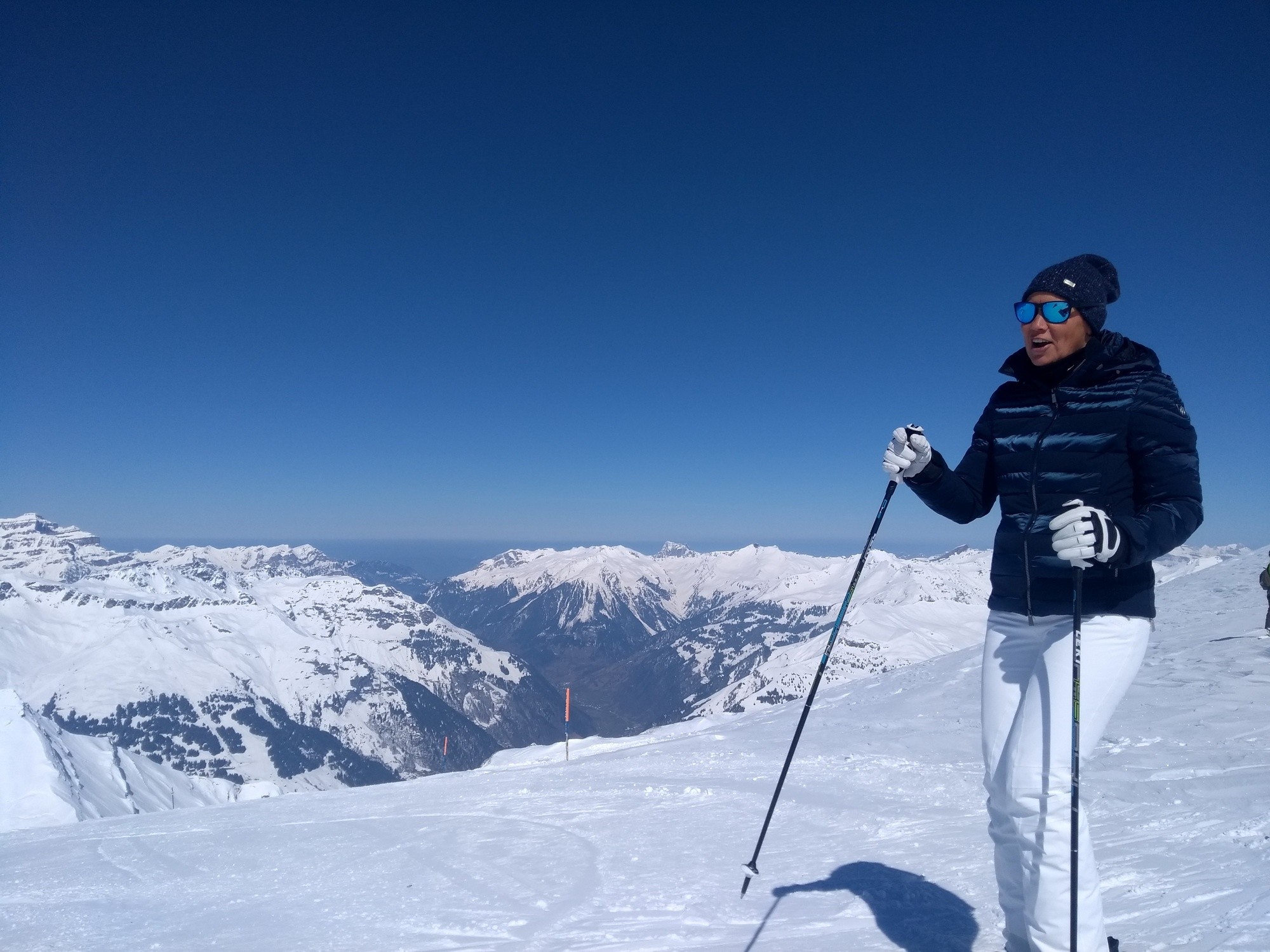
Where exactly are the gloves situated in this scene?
[882,427,932,478]
[1049,499,1121,563]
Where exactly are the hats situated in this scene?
[1022,254,1120,332]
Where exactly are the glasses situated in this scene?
[1014,301,1073,324]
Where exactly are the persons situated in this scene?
[1265,551,1270,636]
[883,254,1204,952]
